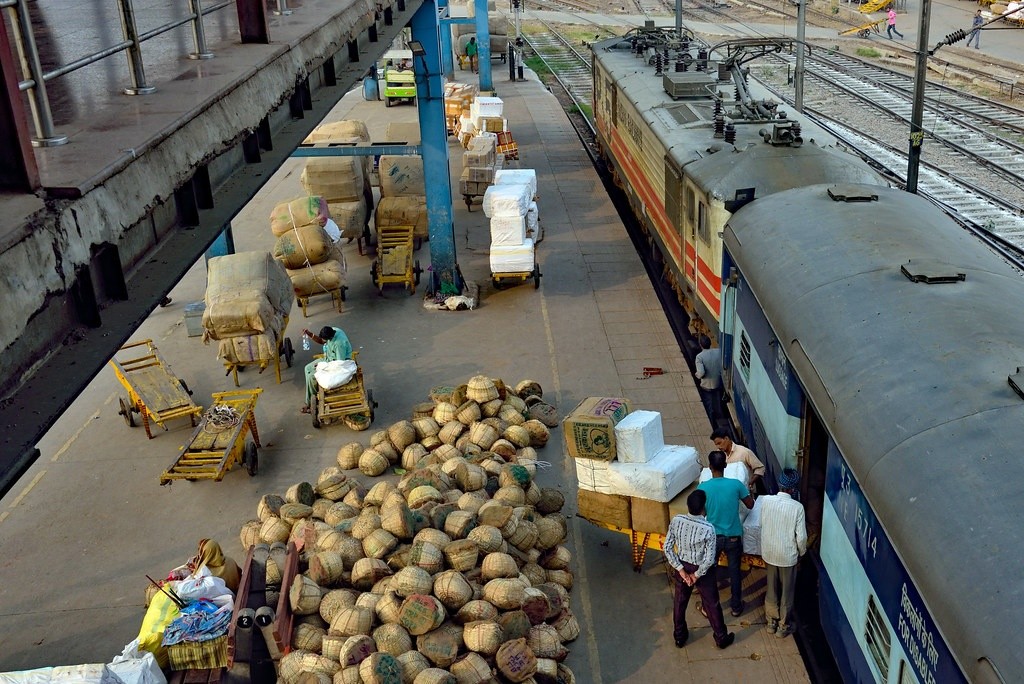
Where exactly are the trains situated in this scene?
[587,19,1024,683]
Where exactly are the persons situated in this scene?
[710,428,766,501]
[664,490,735,649]
[743,467,806,638]
[806,462,821,552]
[401,58,410,70]
[695,333,723,427]
[966,10,983,49]
[299,325,353,413]
[465,37,478,74]
[695,451,754,618]
[886,6,904,41]
[192,537,242,594]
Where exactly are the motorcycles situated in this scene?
[383,49,417,107]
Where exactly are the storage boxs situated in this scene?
[454,96,518,196]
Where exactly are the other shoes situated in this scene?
[301,406,311,414]
[676,630,690,647]
[768,622,796,637]
[720,631,735,650]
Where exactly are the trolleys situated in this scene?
[446,125,520,213]
[223,284,295,388]
[296,236,348,318]
[159,386,264,487]
[310,350,379,429]
[490,195,545,290]
[370,225,425,298]
[836,19,888,39]
[576,505,767,574]
[456,50,507,71]
[107,338,203,440]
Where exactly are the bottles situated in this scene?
[302,332,310,351]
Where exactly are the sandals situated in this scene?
[731,600,746,617]
[694,601,709,618]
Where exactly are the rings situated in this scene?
[750,485,752,488]
